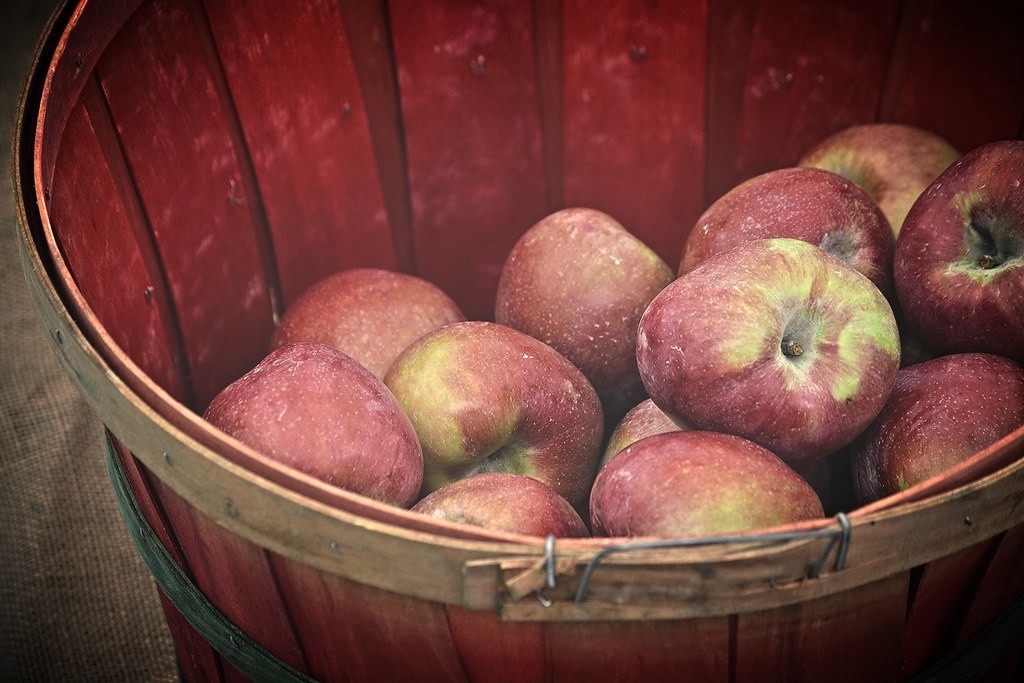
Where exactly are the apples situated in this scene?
[205,126,1024,537]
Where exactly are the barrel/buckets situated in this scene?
[13,0,1022,682]
